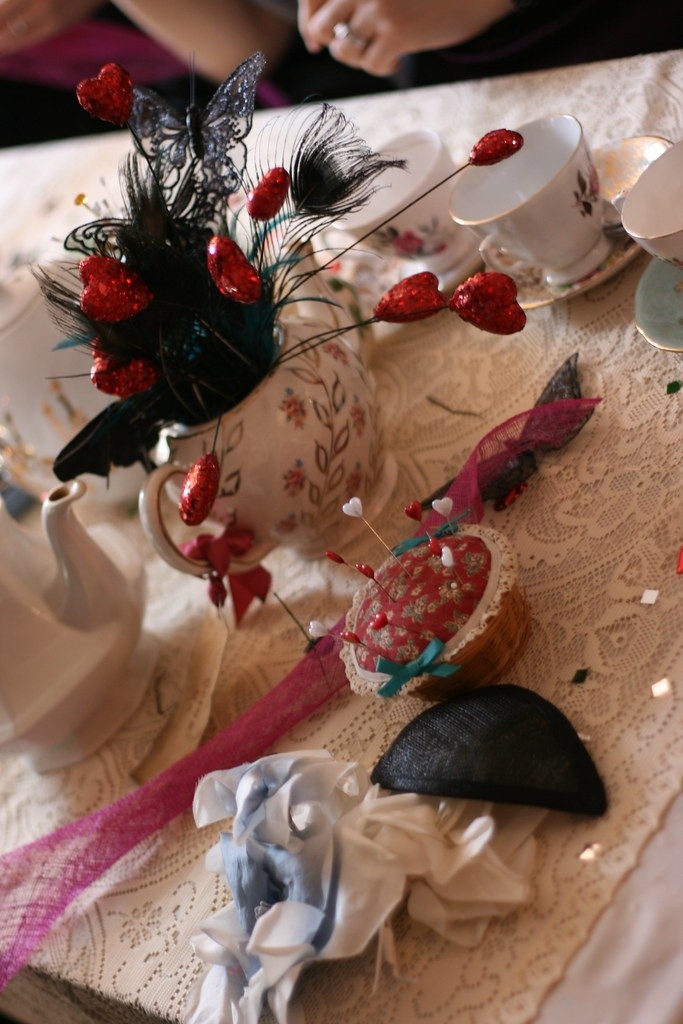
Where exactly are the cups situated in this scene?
[618,136,683,264]
[447,113,612,285]
[322,125,474,280]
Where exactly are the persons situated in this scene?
[102,1,683,103]
[0,2,299,160]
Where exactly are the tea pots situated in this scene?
[1,262,131,471]
[2,476,158,780]
[136,235,400,579]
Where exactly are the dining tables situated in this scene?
[0,50,682,1024]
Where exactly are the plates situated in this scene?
[325,158,480,309]
[634,255,682,354]
[484,132,676,309]
